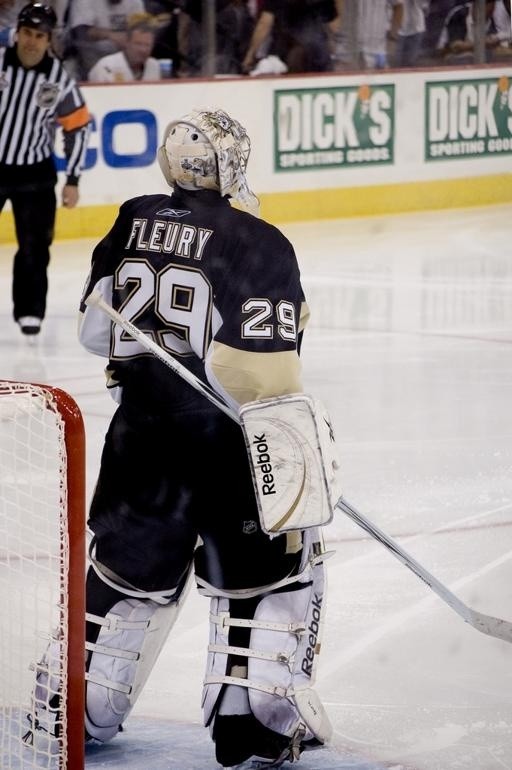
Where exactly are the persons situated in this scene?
[0,2,92,334]
[19,109,343,770]
[1,0,511,85]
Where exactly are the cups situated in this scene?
[159,59,173,79]
[374,51,385,69]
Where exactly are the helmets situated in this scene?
[158,104,251,197]
[17,2,57,33]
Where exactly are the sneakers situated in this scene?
[17,316,40,335]
